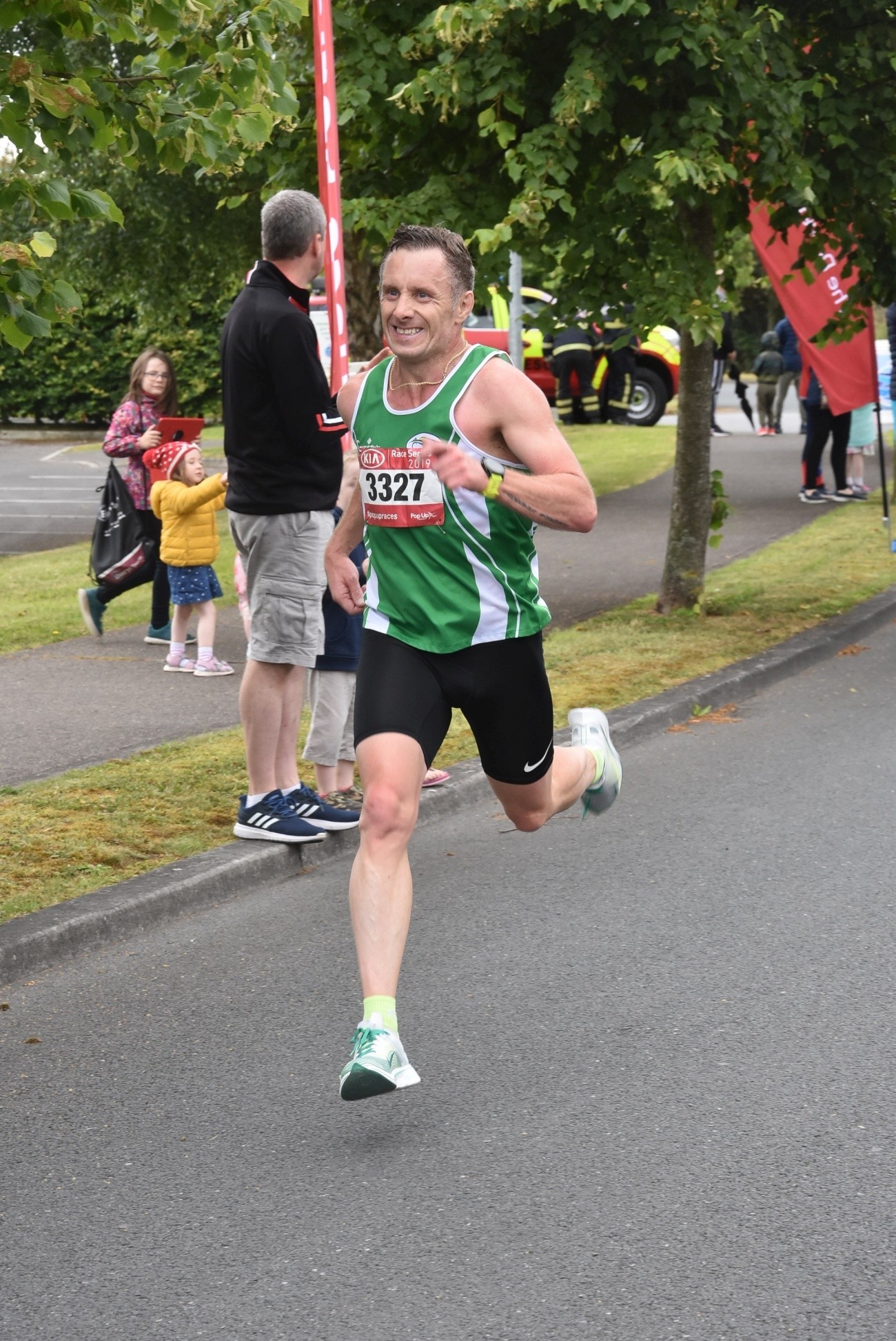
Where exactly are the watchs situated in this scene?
[481,456,505,500]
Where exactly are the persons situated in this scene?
[688,265,896,505]
[325,228,623,1101]
[543,301,648,427]
[78,190,451,843]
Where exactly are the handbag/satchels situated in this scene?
[86,463,155,588]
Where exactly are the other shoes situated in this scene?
[775,425,782,433]
[711,425,732,437]
[800,425,807,433]
[768,427,776,436]
[757,427,767,436]
[848,482,871,494]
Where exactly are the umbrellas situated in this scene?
[728,361,755,430]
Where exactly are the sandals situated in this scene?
[163,653,196,672]
[193,656,235,676]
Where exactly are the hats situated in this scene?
[142,441,203,479]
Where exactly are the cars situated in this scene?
[380,283,682,427]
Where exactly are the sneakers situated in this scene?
[338,1014,422,1102]
[567,707,622,819]
[78,587,107,637]
[322,787,365,810]
[801,491,827,504]
[233,789,328,843]
[798,485,834,498]
[283,781,361,831]
[143,620,197,645]
[422,768,449,786]
[833,490,867,502]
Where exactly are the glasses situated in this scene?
[143,372,170,382]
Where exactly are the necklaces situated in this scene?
[389,340,468,391]
